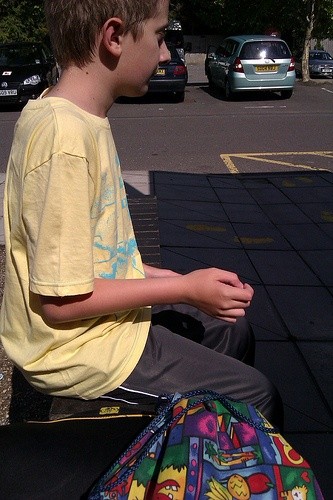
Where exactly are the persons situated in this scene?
[0,0,276,423]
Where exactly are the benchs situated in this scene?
[23,195,165,424]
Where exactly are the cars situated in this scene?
[295,50,333,78]
[204,34,296,100]
[117,46,188,102]
[0,42,60,109]
